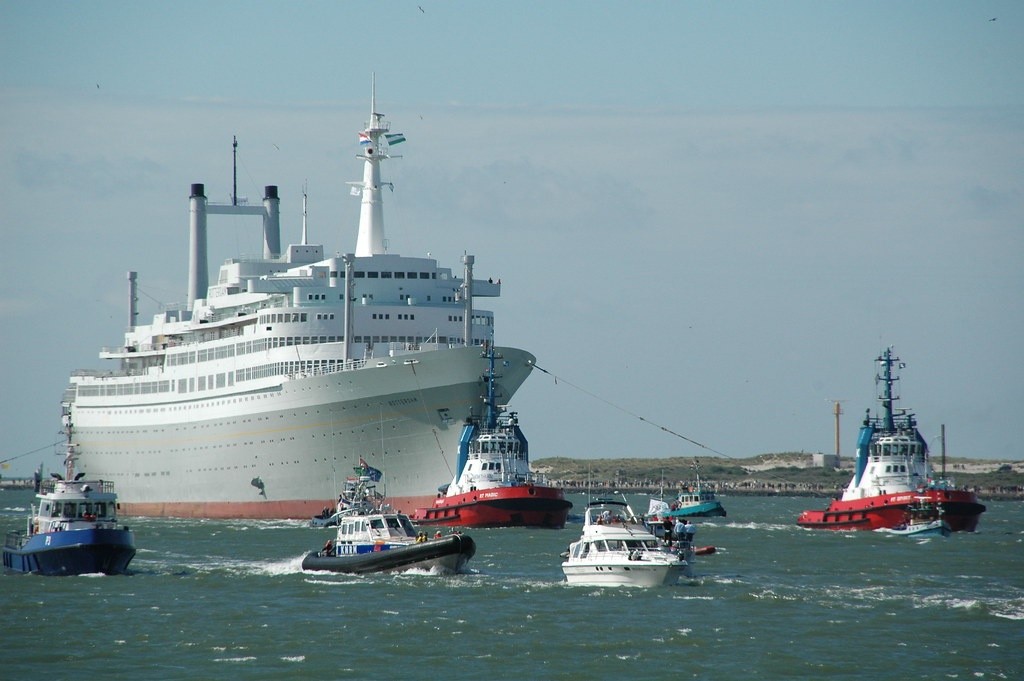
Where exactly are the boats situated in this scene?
[560,462,690,589]
[642,511,718,557]
[880,474,954,537]
[401,326,574,530]
[301,474,478,579]
[795,343,987,533]
[652,457,729,520]
[1,413,138,575]
[308,455,395,528]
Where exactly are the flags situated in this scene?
[359,133,371,145]
[384,133,406,146]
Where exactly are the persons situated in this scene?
[322,540,333,557]
[416,532,428,542]
[663,516,673,546]
[603,510,612,518]
[674,519,696,542]
[520,480,534,486]
[671,504,675,511]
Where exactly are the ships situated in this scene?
[56,70,538,525]
